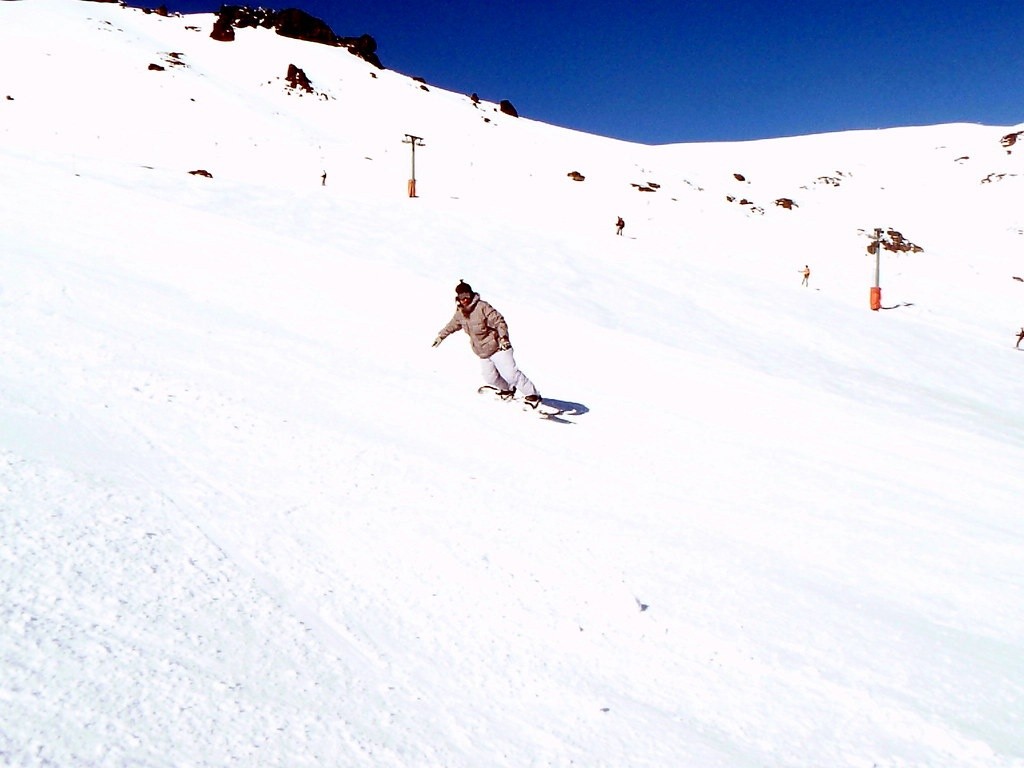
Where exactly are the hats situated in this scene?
[455,280,472,294]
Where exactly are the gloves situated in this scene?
[499,338,511,350]
[431,336,441,348]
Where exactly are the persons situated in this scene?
[801,265,810,287]
[321,169,326,187]
[434,280,543,408]
[1015,328,1024,348]
[615,216,625,236]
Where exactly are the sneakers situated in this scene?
[496,386,517,395]
[525,395,543,401]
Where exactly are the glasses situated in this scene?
[458,293,470,299]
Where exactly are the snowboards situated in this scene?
[478,384,577,418]
